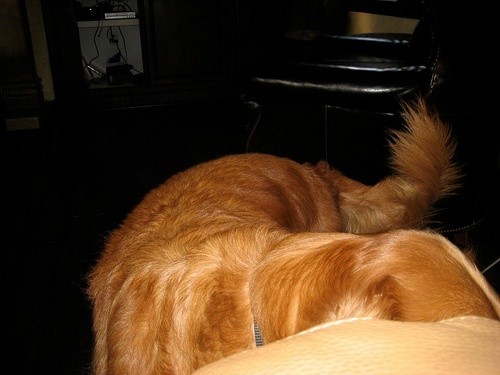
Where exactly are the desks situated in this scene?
[76,71,271,219]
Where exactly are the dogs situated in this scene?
[86,103,500,375]
